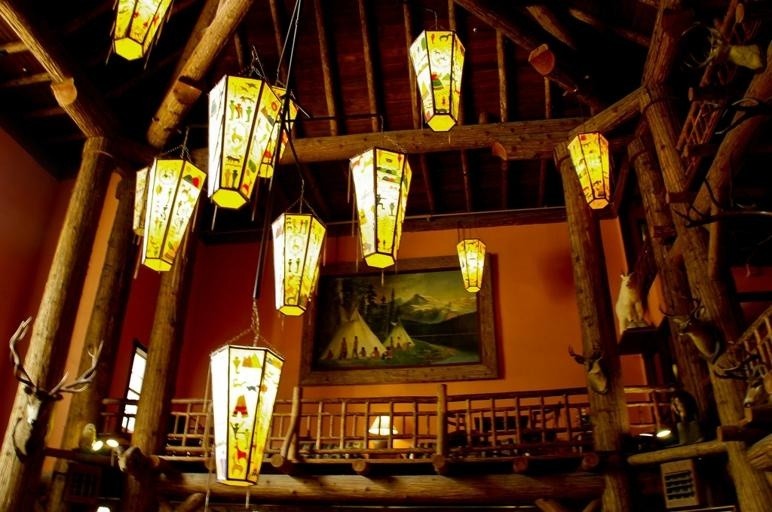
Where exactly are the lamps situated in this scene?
[272,183,327,318]
[132,125,206,272]
[109,0,172,61]
[365,413,400,451]
[208,0,301,209]
[349,113,413,270]
[455,215,486,294]
[567,92,611,210]
[408,6,464,133]
[210,208,286,486]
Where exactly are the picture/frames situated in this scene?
[300,251,502,387]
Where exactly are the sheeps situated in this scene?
[110,444,148,476]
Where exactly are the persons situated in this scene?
[671,390,707,443]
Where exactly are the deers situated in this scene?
[8,316,104,429]
[562,336,612,394]
[654,294,723,363]
[711,345,772,412]
